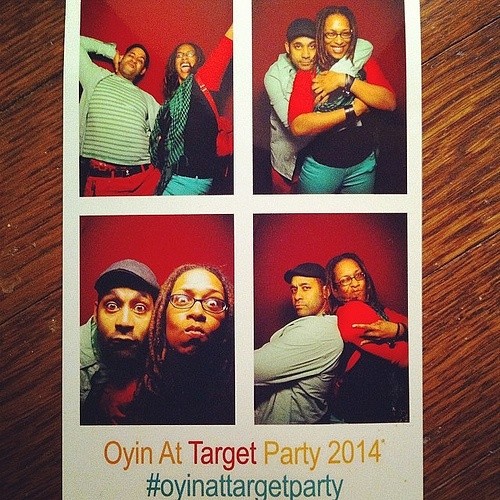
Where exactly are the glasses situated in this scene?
[323,29,353,39]
[168,294,231,314]
[175,50,196,58]
[334,272,366,286]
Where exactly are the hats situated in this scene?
[284,263,326,283]
[94,258,161,298]
[286,19,316,43]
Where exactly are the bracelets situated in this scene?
[344,72,355,90]
[395,322,402,339]
[344,104,358,120]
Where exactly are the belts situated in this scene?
[87,162,149,177]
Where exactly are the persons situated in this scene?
[252,251,409,424]
[78,258,236,425]
[148,21,234,195]
[263,5,397,194]
[79,33,163,196]
